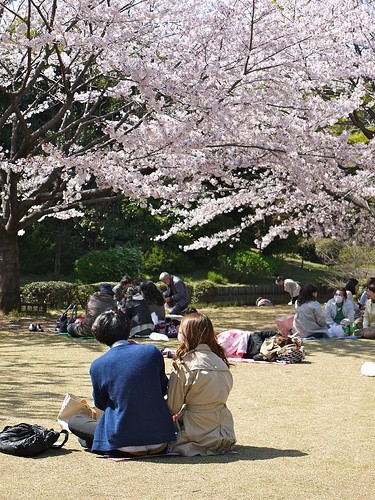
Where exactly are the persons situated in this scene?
[67,310,172,459]
[67,272,190,341]
[160,313,236,457]
[276,276,375,339]
[205,329,301,362]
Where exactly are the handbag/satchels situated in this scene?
[55,304,78,332]
[274,315,294,337]
[0,423,69,456]
[328,325,345,338]
[181,306,197,316]
[57,393,104,435]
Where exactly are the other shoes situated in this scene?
[28,323,43,332]
[161,347,173,358]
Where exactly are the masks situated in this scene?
[334,296,343,304]
